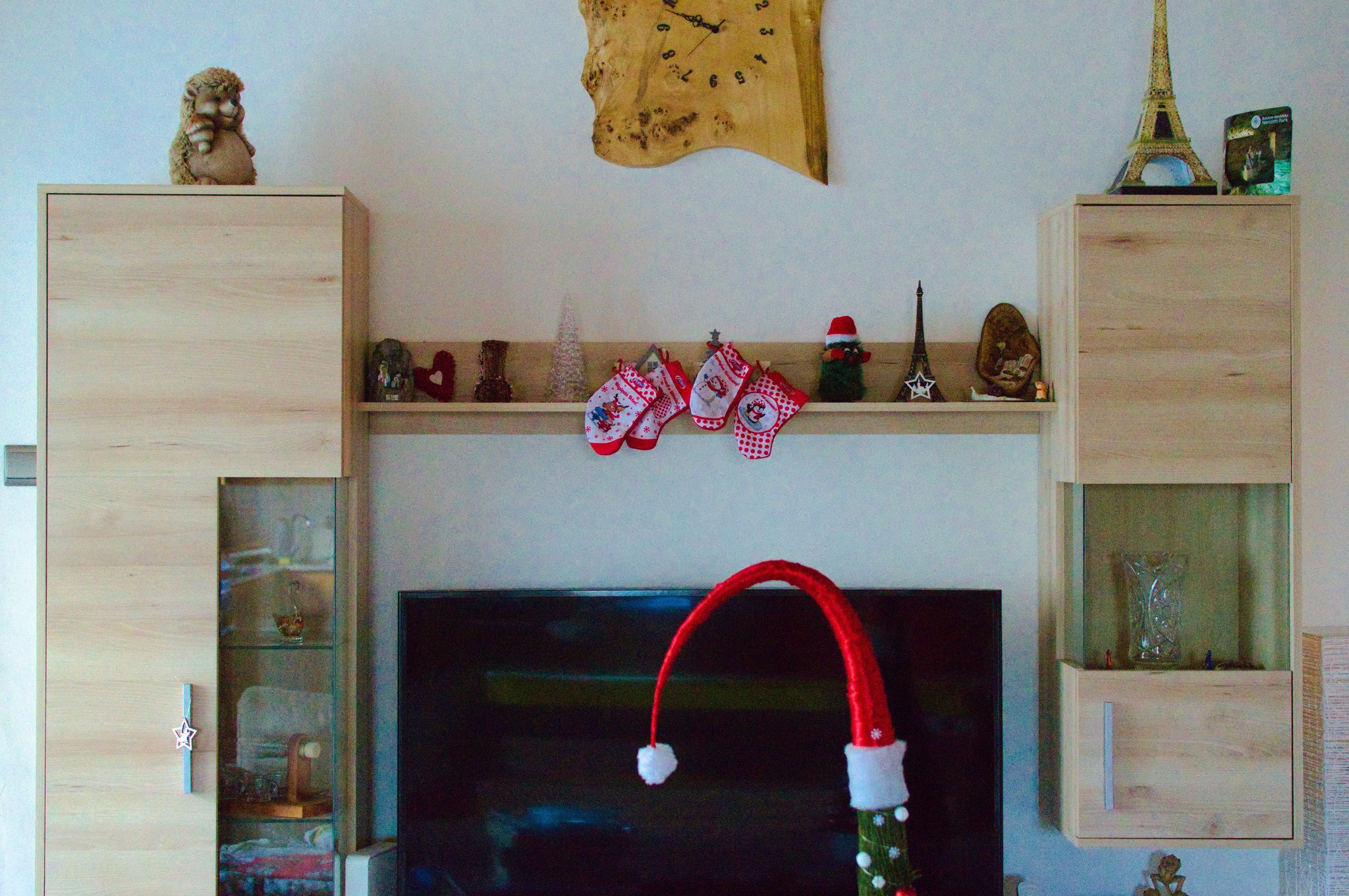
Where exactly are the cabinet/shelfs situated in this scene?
[35,185,372,896]
[1039,195,1304,850]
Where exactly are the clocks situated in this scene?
[578,0,828,187]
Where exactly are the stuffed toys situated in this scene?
[814,315,871,402]
[168,67,256,184]
